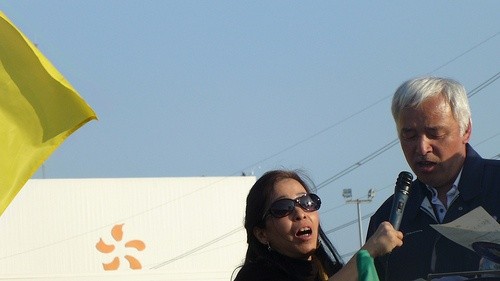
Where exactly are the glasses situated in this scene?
[262,193,321,221]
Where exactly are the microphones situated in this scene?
[385,171,413,257]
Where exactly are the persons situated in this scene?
[230,170,403,281]
[366,77,500,281]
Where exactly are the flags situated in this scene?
[0,12,99,218]
